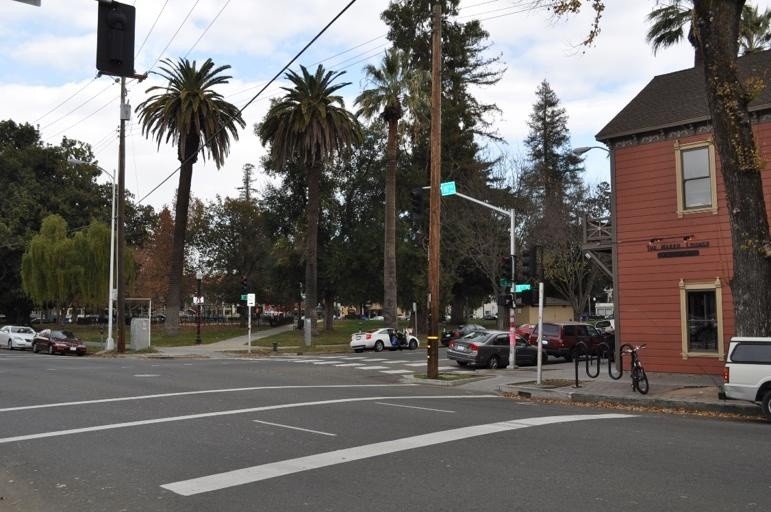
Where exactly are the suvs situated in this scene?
[718,336,771,419]
[527,321,605,361]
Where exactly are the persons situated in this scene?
[392,328,404,351]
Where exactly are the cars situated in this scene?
[516,324,535,338]
[30,308,113,325]
[345,309,413,321]
[349,328,420,352]
[1,325,38,350]
[442,324,485,347]
[446,330,547,369]
[31,329,86,357]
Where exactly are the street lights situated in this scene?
[194,268,204,346]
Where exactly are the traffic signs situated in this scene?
[511,284,531,294]
[498,278,512,287]
[247,293,256,307]
[241,295,247,300]
[440,181,457,196]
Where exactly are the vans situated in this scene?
[595,319,614,336]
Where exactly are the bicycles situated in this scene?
[621,342,650,395]
